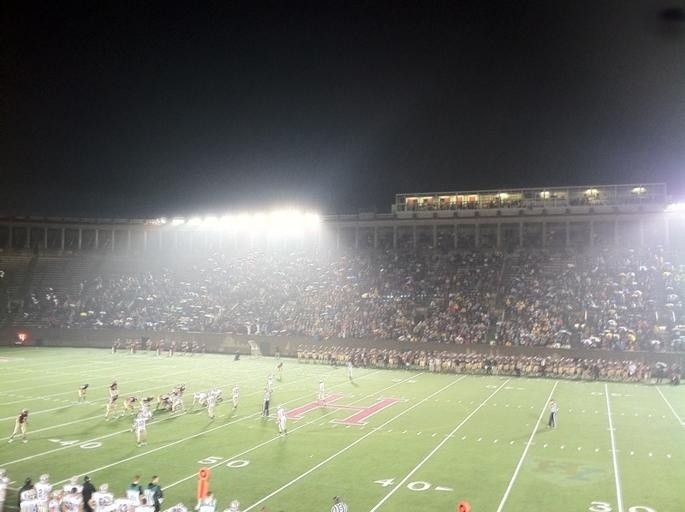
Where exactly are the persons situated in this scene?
[273,404,290,437]
[457,499,473,512]
[73,378,247,445]
[260,386,271,422]
[1,461,243,512]
[7,409,33,444]
[391,188,671,214]
[327,495,349,512]
[318,374,328,401]
[546,398,561,430]
